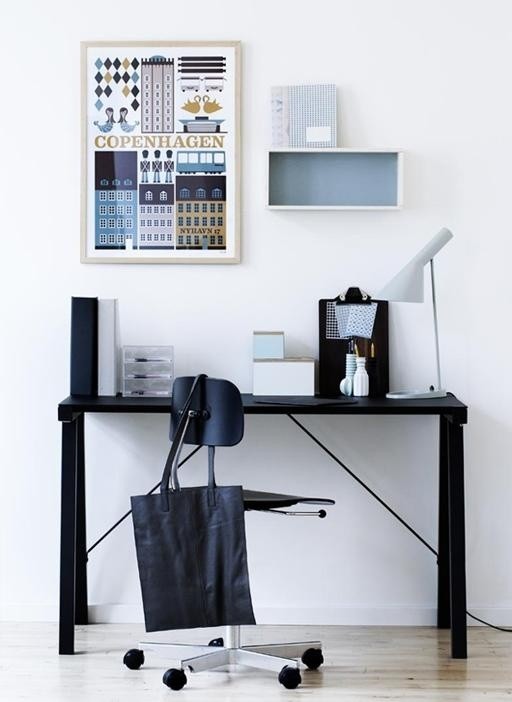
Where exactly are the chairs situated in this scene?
[123,376,335,689]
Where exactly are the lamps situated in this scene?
[368,224,454,400]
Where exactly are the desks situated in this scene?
[57,392,468,659]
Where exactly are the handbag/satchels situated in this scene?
[130,485,257,632]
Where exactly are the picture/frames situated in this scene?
[79,39,242,266]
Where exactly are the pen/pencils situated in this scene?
[348,338,374,357]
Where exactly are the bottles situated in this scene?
[353,357,370,397]
